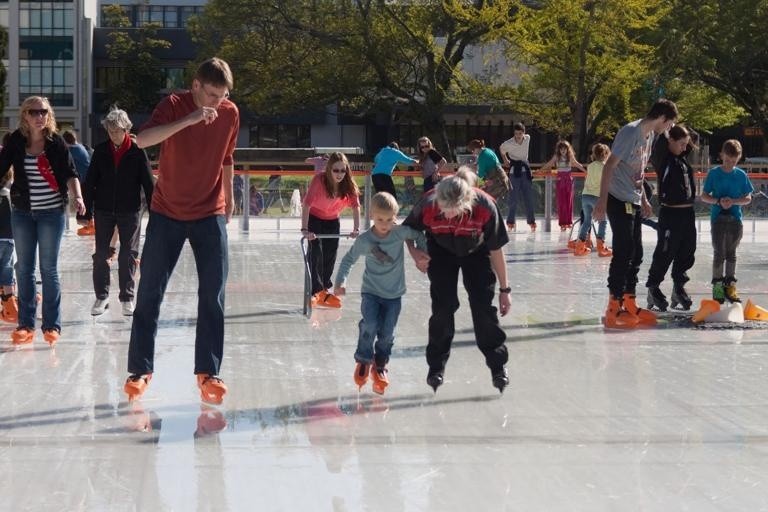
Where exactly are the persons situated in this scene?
[539,140,587,230]
[125,57,243,412]
[699,137,753,304]
[402,167,510,391]
[334,192,428,395]
[300,153,359,309]
[592,97,679,329]
[569,142,612,258]
[230,162,292,218]
[0,95,157,347]
[647,124,698,311]
[466,137,511,203]
[306,153,329,175]
[499,121,538,231]
[369,140,418,199]
[415,137,447,191]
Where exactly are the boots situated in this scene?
[594,238,613,257]
[566,236,593,250]
[574,239,591,256]
[670,285,694,311]
[711,278,726,305]
[623,293,657,327]
[604,294,640,331]
[646,285,670,313]
[724,278,743,304]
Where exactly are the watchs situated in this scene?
[498,287,510,294]
[353,227,359,232]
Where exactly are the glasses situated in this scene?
[329,167,348,175]
[201,85,231,105]
[24,108,49,117]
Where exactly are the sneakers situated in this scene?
[36,292,42,305]
[426,368,445,394]
[78,218,96,236]
[313,290,342,310]
[42,327,61,348]
[90,297,111,324]
[195,406,227,438]
[0,293,19,324]
[530,222,537,231]
[197,374,228,406]
[124,372,153,404]
[11,326,36,349]
[353,360,371,393]
[370,363,390,396]
[507,223,516,231]
[559,223,573,232]
[120,301,135,322]
[491,366,510,393]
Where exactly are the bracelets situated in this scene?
[300,228,309,232]
